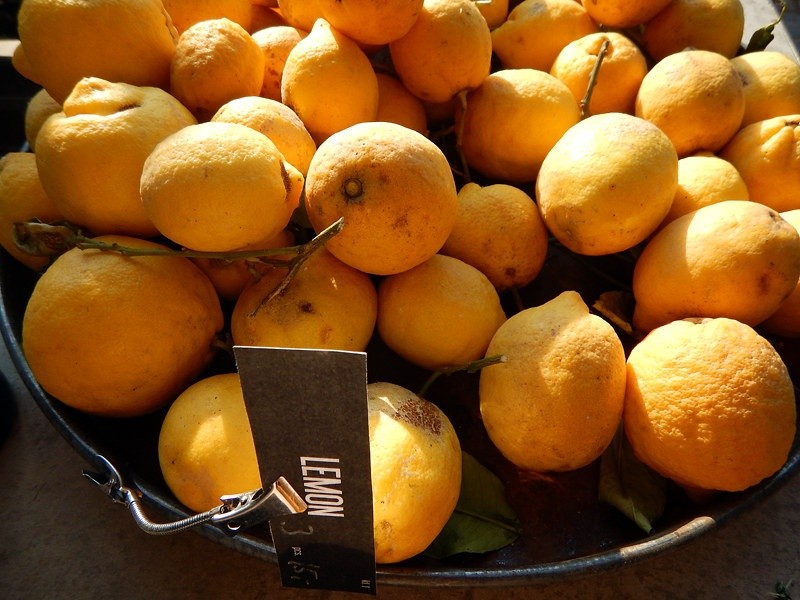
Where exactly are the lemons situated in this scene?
[0,0,800,567]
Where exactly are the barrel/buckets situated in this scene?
[0,73,800,588]
